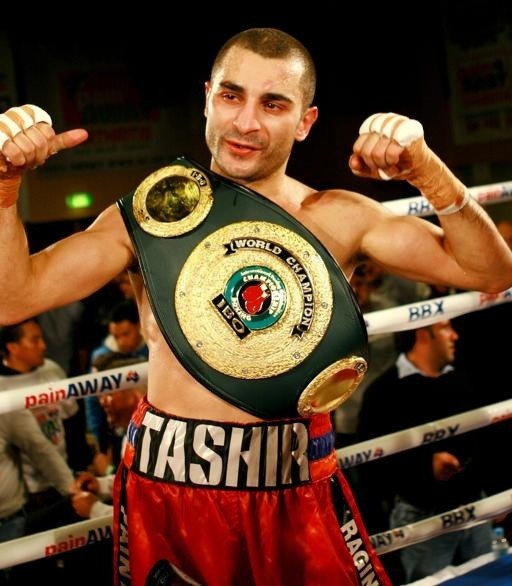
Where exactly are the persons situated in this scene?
[354,317,496,579]
[0,268,150,586]
[0,28,512,586]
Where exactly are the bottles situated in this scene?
[492,528,508,560]
